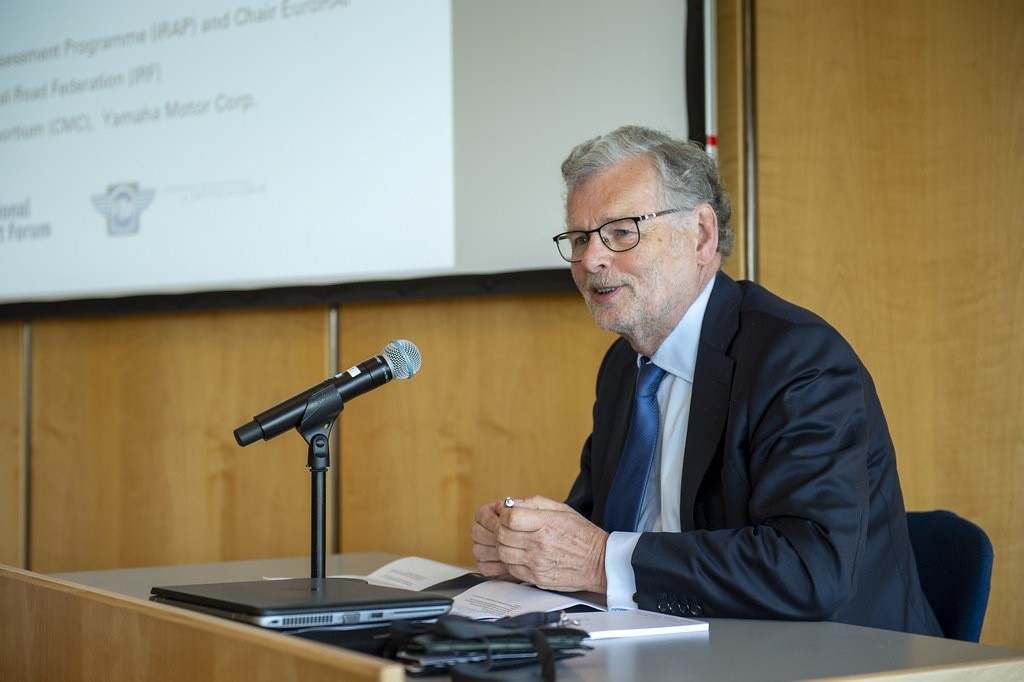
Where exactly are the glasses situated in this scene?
[552,207,696,263]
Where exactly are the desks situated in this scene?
[44,551,1024,682]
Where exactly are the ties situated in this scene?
[602,356,668,533]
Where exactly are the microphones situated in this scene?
[232,338,422,449]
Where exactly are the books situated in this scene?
[566,608,709,639]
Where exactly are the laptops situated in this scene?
[148,576,456,638]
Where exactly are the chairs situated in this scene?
[908,510,994,645]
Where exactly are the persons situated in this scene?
[470,125,945,638]
